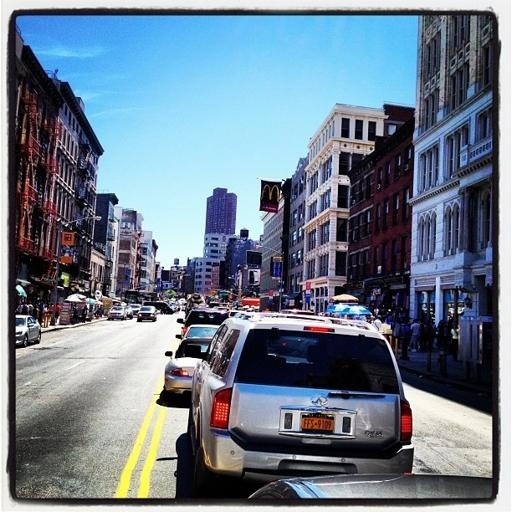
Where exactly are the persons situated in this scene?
[15,301,104,328]
[323,306,459,362]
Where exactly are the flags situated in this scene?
[259,179,281,213]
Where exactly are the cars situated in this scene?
[15,314,42,347]
[107,298,174,322]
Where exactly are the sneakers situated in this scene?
[408,345,422,352]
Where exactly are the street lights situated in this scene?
[226,263,258,288]
[121,261,146,298]
[255,244,285,312]
[49,215,102,326]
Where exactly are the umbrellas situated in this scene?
[64,293,104,307]
[331,293,360,302]
